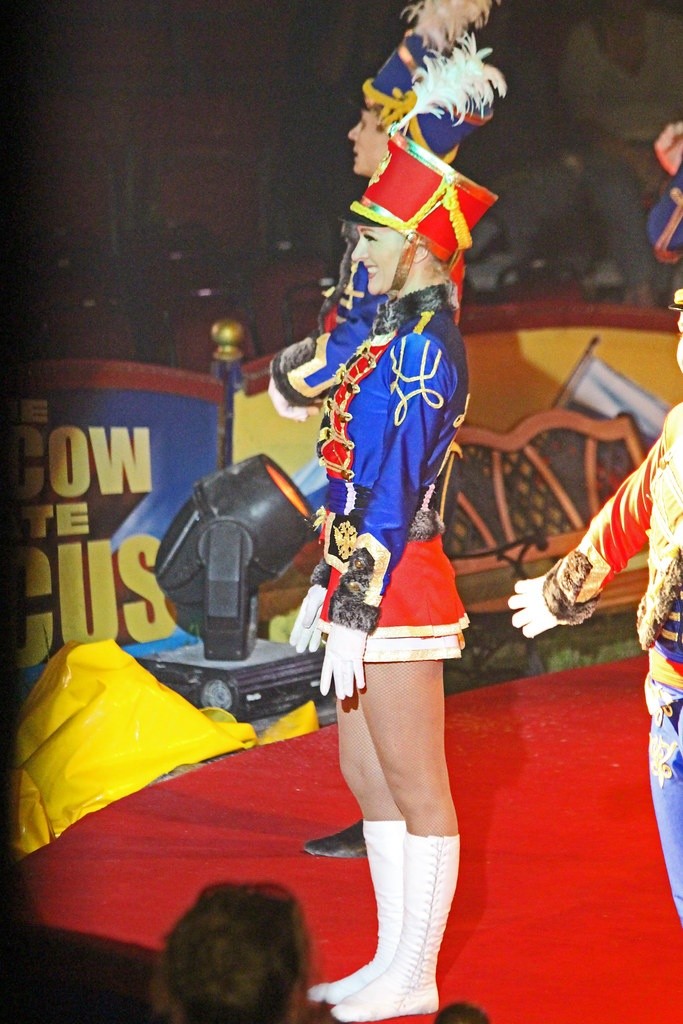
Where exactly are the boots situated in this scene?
[330,831,461,1022]
[324,821,408,1005]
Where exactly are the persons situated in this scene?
[265,1,509,1023]
[509,286,682,922]
[646,120,682,313]
[162,876,316,1023]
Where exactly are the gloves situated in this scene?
[320,621,369,698]
[289,584,326,653]
[508,575,560,638]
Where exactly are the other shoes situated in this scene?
[304,818,367,857]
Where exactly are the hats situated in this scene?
[362,0,502,164]
[349,132,500,262]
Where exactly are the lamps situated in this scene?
[132,452,331,721]
